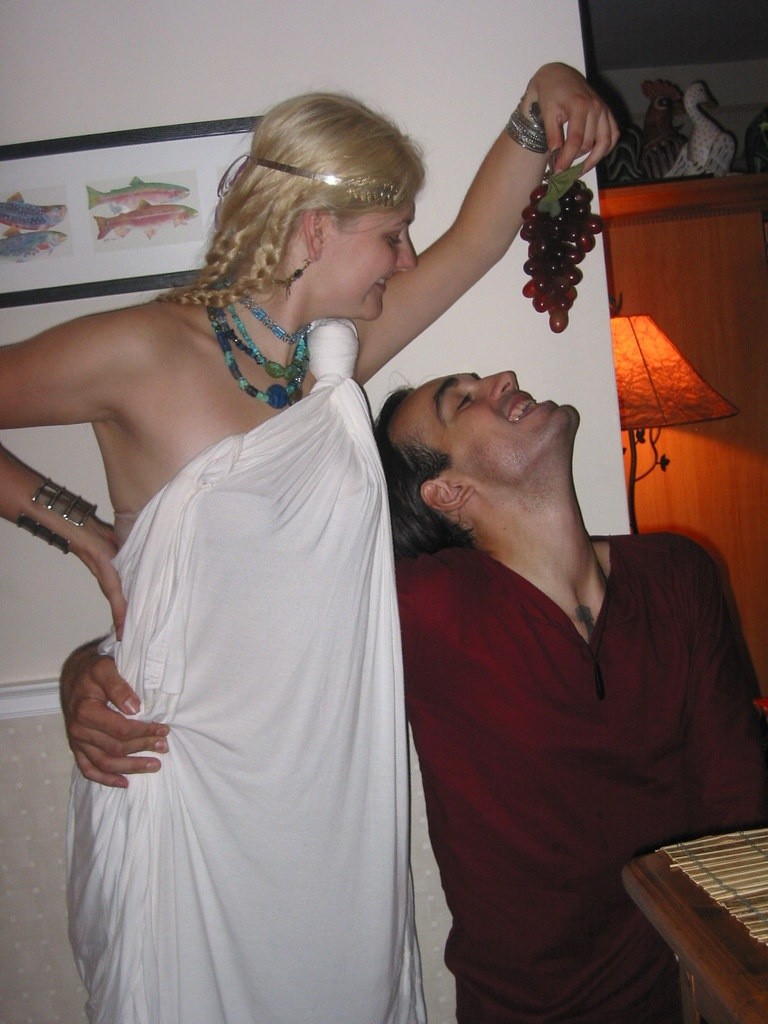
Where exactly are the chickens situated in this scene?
[597,78,684,189]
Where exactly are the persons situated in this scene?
[58,370,768,1024]
[0,60,618,1024]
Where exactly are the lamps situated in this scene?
[610,291,740,537]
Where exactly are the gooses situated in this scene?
[663,78,736,180]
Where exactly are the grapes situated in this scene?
[520,170,602,336]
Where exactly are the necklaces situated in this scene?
[206,280,310,409]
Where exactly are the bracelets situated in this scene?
[16,478,97,554]
[505,102,548,154]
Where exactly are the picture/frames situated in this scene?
[0,114,263,309]
